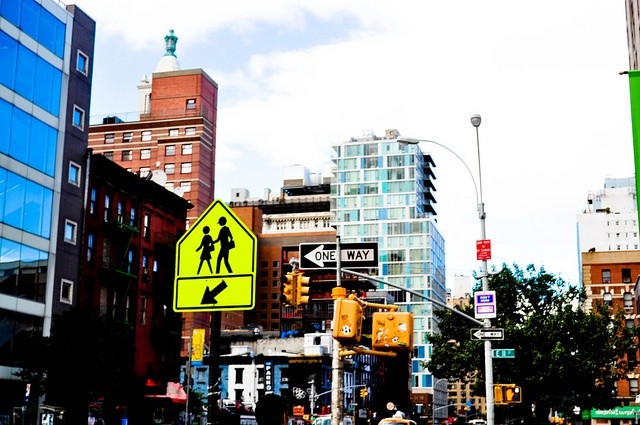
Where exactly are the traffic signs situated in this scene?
[173,198,260,312]
[470,328,505,340]
[474,290,496,319]
[298,241,379,271]
[492,348,516,359]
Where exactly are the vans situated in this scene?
[312,415,354,425]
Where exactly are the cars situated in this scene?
[378,415,416,425]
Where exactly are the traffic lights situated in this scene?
[283,271,311,305]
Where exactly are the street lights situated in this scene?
[396,112,495,425]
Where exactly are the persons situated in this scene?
[255,393,287,425]
